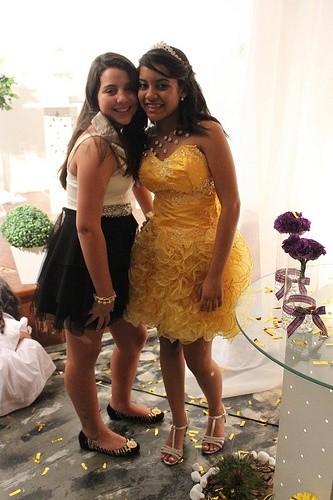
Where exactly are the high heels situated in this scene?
[161,412,190,465]
[201,402,226,453]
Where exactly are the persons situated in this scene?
[123,40,251,466]
[0,277,56,416]
[31,52,165,457]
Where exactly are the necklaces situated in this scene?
[142,123,200,157]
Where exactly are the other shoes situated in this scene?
[79,431,140,457]
[107,403,164,422]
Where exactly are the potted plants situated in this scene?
[1,205,56,283]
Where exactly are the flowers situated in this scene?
[275,212,325,282]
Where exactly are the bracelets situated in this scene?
[92,292,117,304]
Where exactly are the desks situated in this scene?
[232,264,333,500]
